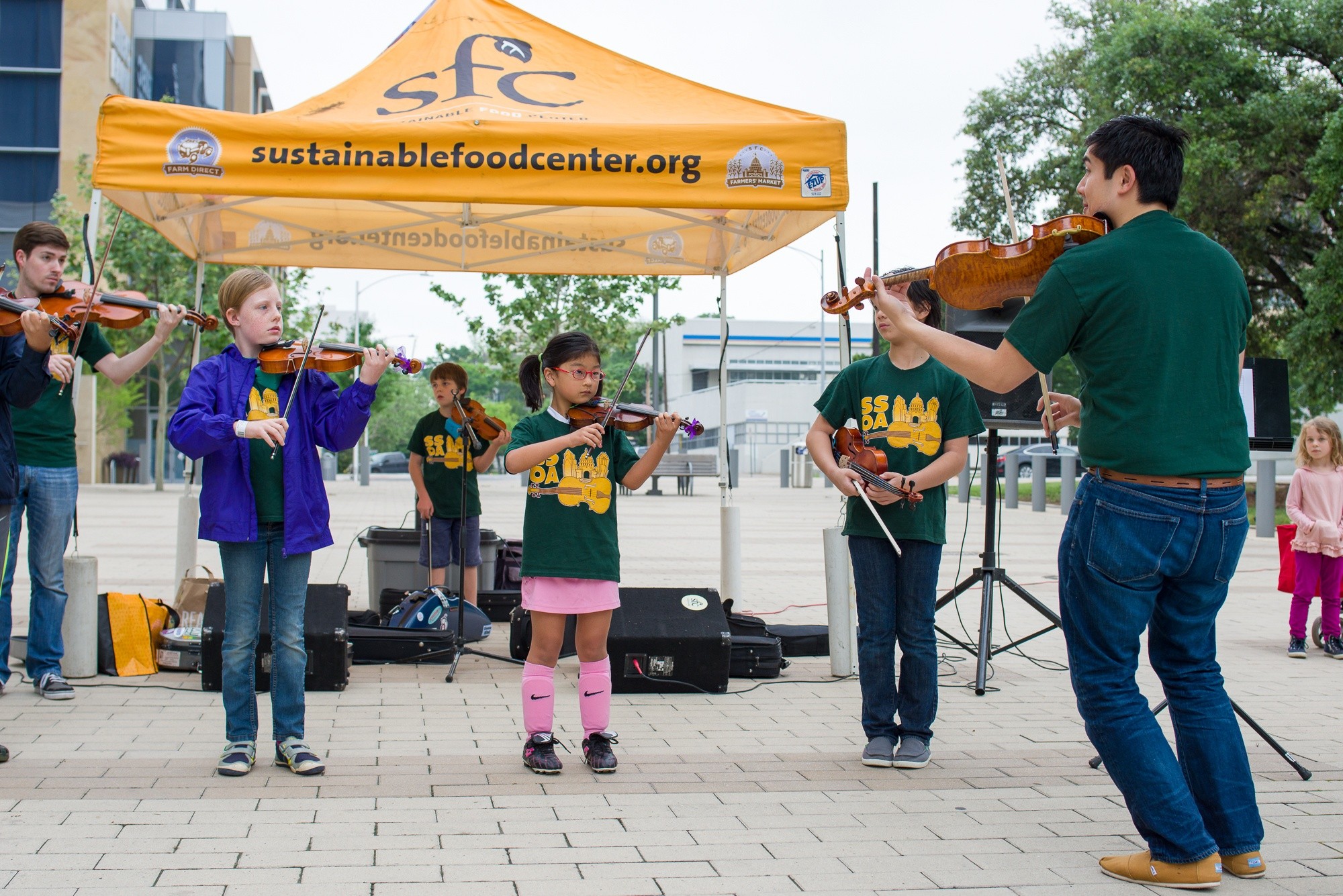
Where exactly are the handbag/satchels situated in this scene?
[98,592,180,677]
[1275,524,1343,598]
[172,564,225,629]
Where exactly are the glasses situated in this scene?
[553,367,607,381]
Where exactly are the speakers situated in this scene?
[606,588,732,694]
[944,298,1049,429]
[201,583,351,693]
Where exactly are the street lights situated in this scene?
[353,273,434,484]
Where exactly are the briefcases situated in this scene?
[764,623,831,657]
[198,582,352,691]
[380,588,418,619]
[723,599,767,636]
[508,604,577,665]
[346,614,456,666]
[729,635,790,679]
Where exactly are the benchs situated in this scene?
[620,454,717,496]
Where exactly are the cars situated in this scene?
[997,443,1087,478]
[370,452,411,474]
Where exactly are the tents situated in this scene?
[81,0,859,677]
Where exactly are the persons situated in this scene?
[165,266,394,774]
[0,222,185,764]
[806,267,985,769]
[502,333,679,774]
[865,119,1266,889]
[1285,416,1343,660]
[408,361,512,608]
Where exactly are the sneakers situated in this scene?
[893,737,933,769]
[521,731,572,774]
[217,740,257,776]
[274,735,326,775]
[1287,635,1309,658]
[34,672,75,700]
[1099,850,1223,889]
[1222,850,1266,879]
[1324,634,1343,659]
[862,736,894,768]
[580,729,619,772]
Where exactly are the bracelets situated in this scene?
[235,419,249,438]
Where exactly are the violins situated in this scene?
[832,425,924,512]
[820,213,1112,322]
[450,396,507,441]
[36,279,219,333]
[256,338,423,376]
[566,396,704,437]
[0,287,81,344]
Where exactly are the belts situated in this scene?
[1088,467,1244,489]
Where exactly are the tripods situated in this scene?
[394,389,527,682]
[1090,357,1312,780]
[934,428,1061,695]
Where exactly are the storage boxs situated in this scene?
[358,526,502,616]
